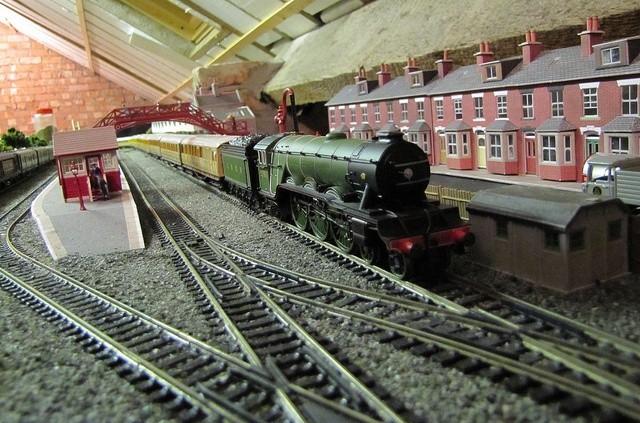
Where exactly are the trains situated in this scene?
[0,144,56,182]
[116,132,474,280]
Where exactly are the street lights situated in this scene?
[70,160,87,211]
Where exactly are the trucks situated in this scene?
[582,154,640,209]
